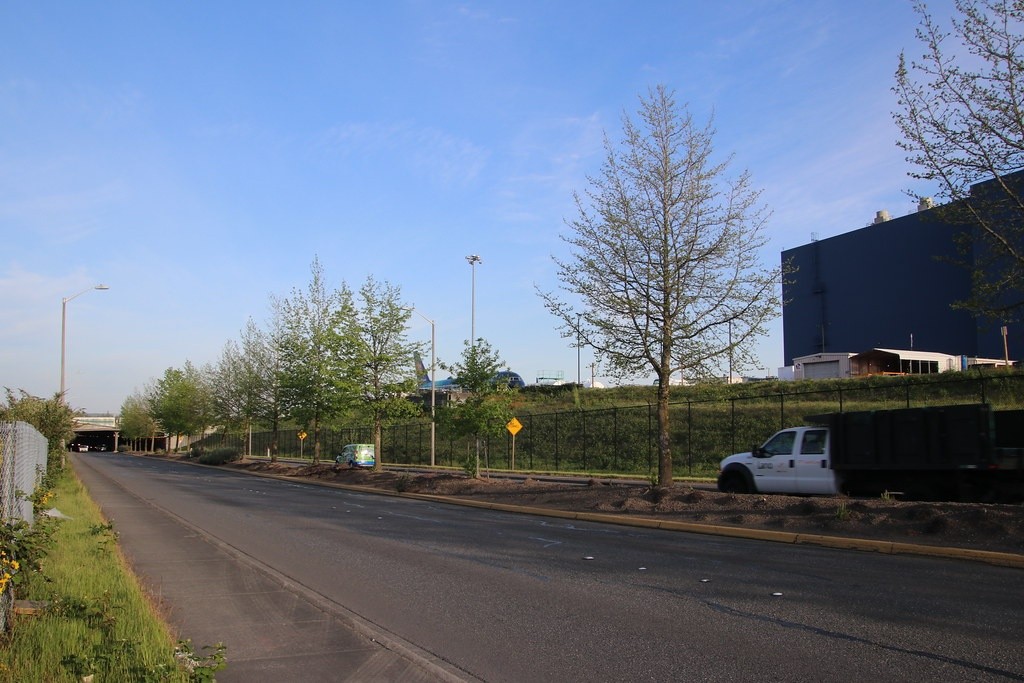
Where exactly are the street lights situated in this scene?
[57,284,109,471]
[400,306,436,467]
[465,253,483,400]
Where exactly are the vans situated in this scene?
[335,444,375,467]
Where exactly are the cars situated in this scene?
[78,444,88,453]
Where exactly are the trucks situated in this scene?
[717,404,1024,502]
